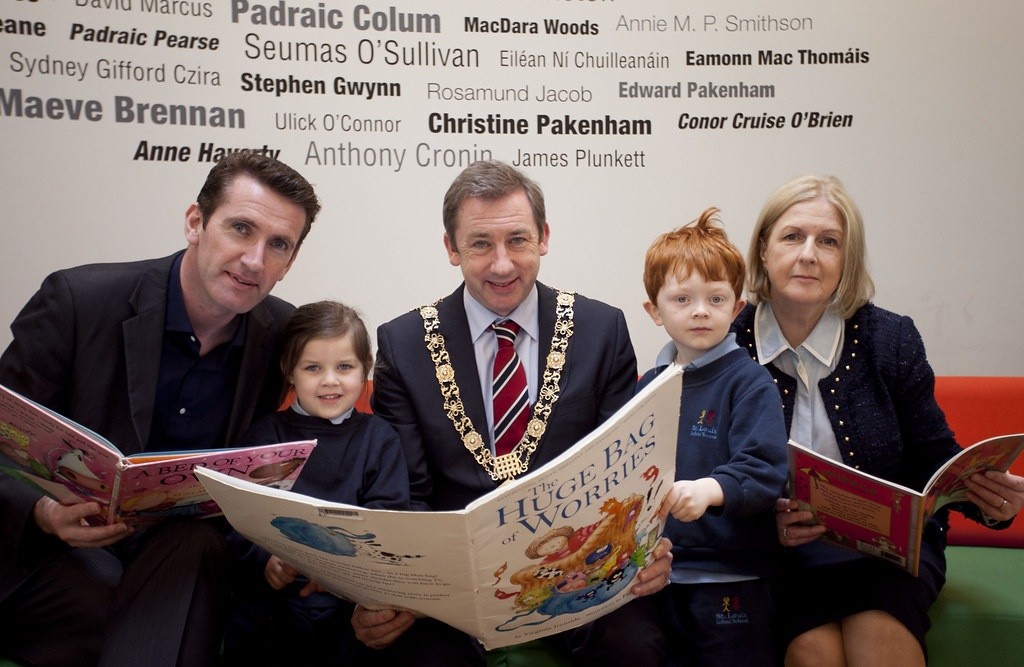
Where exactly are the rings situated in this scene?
[783,528,789,539]
[997,500,1007,511]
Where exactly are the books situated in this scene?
[193,364,688,650]
[2,384,319,526]
[784,432,1024,577]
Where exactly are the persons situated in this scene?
[0,152,323,667]
[731,173,1024,667]
[349,159,676,666]
[260,300,405,667]
[634,207,789,667]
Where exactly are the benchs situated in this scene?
[274,376,1024,667]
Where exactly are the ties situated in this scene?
[491,319,532,457]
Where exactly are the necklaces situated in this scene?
[419,292,575,491]
